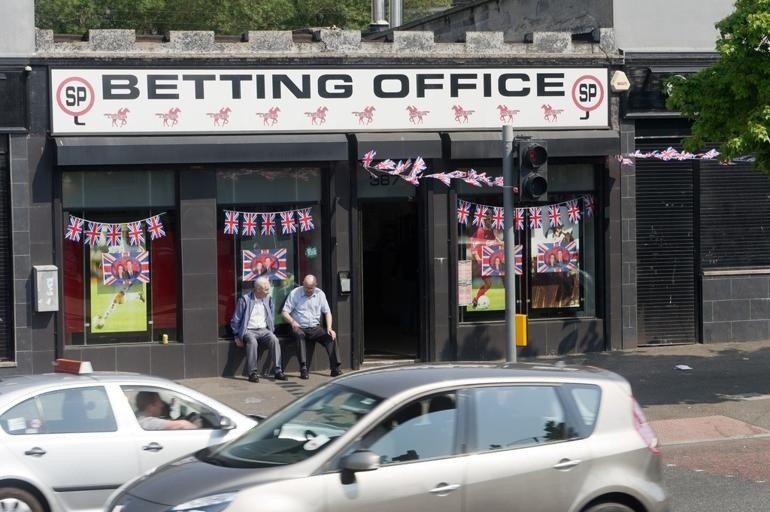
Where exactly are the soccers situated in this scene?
[478,296,489,308]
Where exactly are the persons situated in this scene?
[548,254,557,267]
[230,278,287,382]
[254,261,264,275]
[264,257,275,274]
[125,260,139,279]
[114,264,127,279]
[493,256,502,271]
[134,391,203,429]
[281,274,343,379]
[556,250,568,265]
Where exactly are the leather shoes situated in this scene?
[331,371,342,377]
[275,372,288,380]
[301,369,309,379]
[249,373,259,382]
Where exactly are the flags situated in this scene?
[224,207,315,240]
[102,251,150,286]
[361,150,596,229]
[482,244,524,275]
[613,147,755,167]
[536,241,578,273]
[241,248,288,281]
[63,215,167,249]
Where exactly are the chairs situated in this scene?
[396,395,452,425]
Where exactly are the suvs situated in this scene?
[107,360,666,512]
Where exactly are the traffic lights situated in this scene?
[517,140,549,204]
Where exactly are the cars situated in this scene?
[0,358,263,511]
